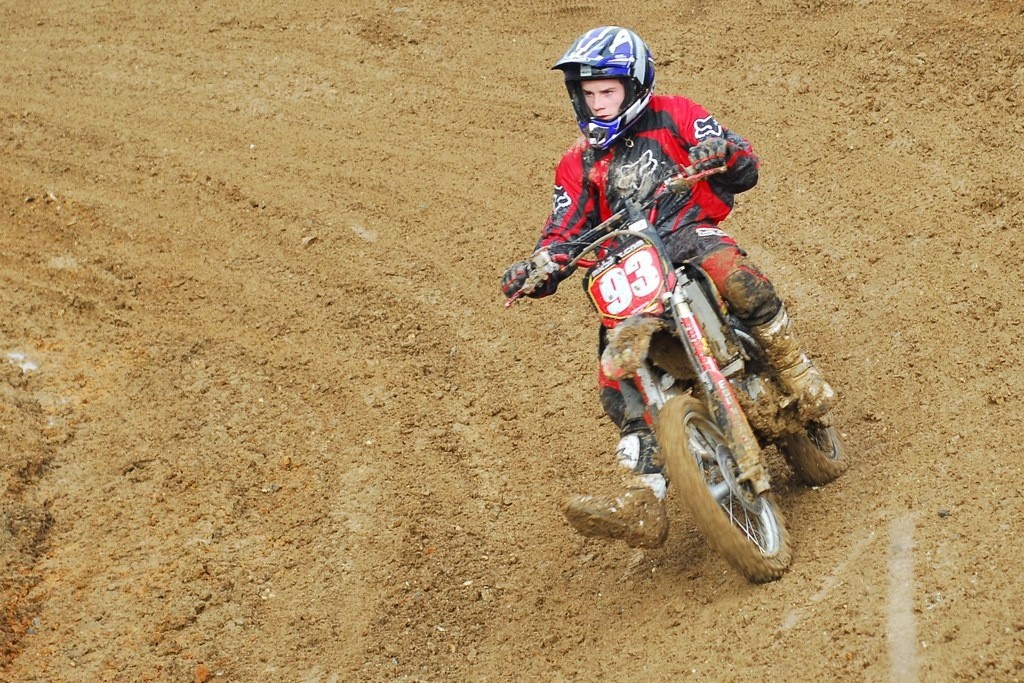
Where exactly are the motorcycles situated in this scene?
[502,158,849,584]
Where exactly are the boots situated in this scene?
[745,302,839,418]
[560,433,672,549]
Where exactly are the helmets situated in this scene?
[551,25,655,152]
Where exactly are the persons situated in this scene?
[499,26,837,548]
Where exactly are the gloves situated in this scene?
[499,261,537,299]
[689,138,734,173]
